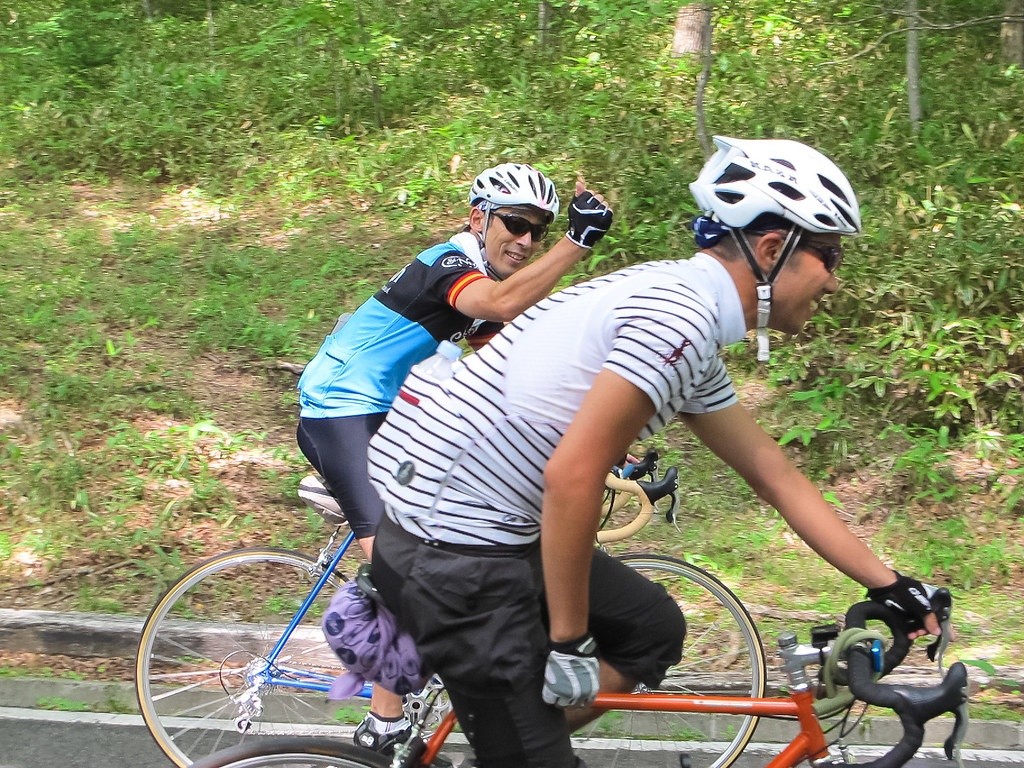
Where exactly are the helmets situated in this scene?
[469,162,560,227]
[688,135,862,236]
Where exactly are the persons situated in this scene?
[296,166,615,768]
[366,135,960,767]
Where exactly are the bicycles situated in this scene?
[183,563,972,768]
[134,449,683,768]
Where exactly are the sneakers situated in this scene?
[353,715,454,768]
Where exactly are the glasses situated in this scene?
[490,210,550,242]
[744,229,844,274]
